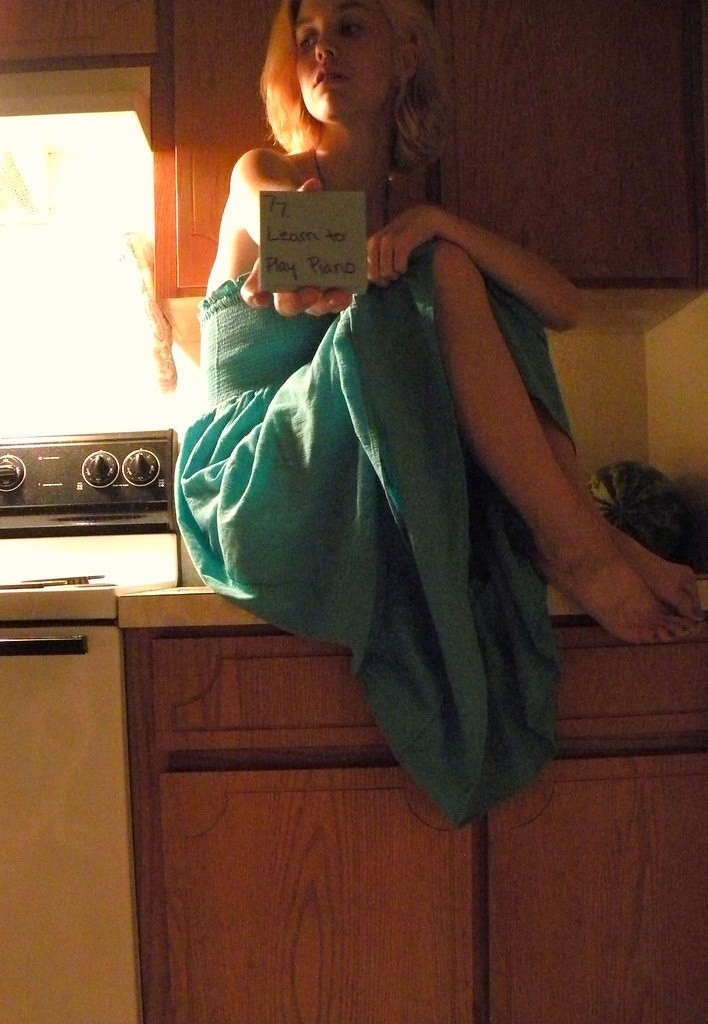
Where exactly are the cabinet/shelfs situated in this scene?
[150,0,708,333]
[124,585,708,1024]
[0,0,160,74]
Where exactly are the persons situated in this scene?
[174,0,708,648]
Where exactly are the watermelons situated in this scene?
[584,460,683,558]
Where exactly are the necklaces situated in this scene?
[311,144,392,234]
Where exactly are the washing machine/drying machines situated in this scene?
[0,429,180,1024]
